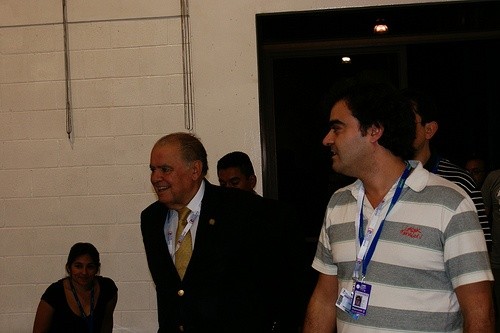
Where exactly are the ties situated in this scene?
[174,207,193,281]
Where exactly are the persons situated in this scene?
[141,133,260,333]
[33,243,118,333]
[304,82,496,333]
[218,90,500,333]
